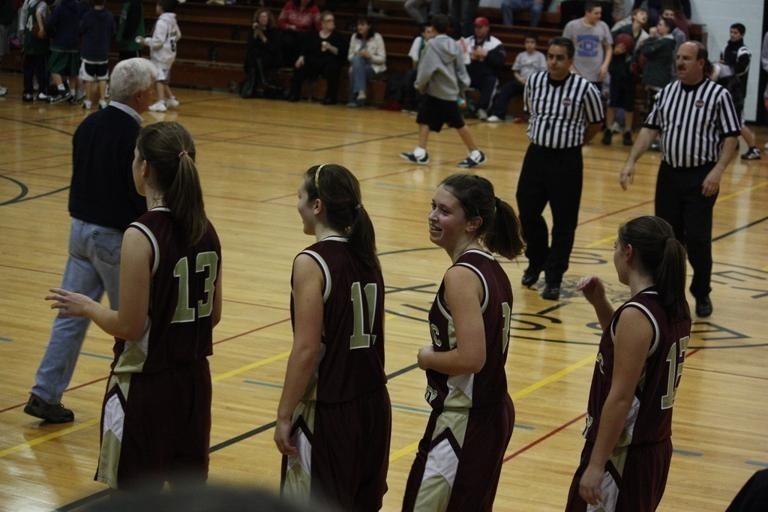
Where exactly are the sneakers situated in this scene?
[521,258,548,287]
[456,150,488,169]
[601,129,633,146]
[742,146,761,160]
[148,95,181,112]
[477,108,529,122]
[696,291,712,317]
[400,149,430,164]
[24,85,110,109]
[345,97,367,107]
[23,392,75,422]
[542,278,561,300]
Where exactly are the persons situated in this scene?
[0,0,185,115]
[564,216,689,511]
[239,1,767,169]
[620,42,740,317]
[515,38,603,301]
[400,173,527,512]
[273,165,391,510]
[44,126,224,496]
[20,59,167,420]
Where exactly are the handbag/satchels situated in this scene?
[25,7,50,36]
